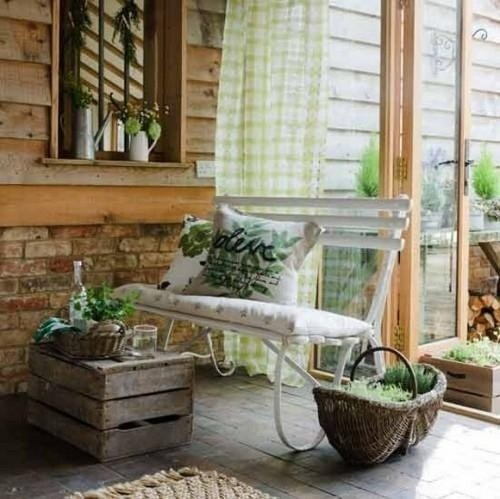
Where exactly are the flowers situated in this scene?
[114,100,172,139]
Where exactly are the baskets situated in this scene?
[311,346,448,469]
[52,305,128,360]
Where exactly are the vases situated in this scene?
[125,130,151,162]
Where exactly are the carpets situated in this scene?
[57,463,279,499]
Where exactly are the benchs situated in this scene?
[101,190,415,451]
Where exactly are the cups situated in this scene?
[132,324,158,357]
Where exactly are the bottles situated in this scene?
[67,260,88,324]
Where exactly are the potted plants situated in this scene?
[423,339,500,415]
[357,138,500,238]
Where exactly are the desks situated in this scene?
[364,224,499,308]
[25,340,199,461]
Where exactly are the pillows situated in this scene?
[150,208,213,292]
[182,203,325,312]
